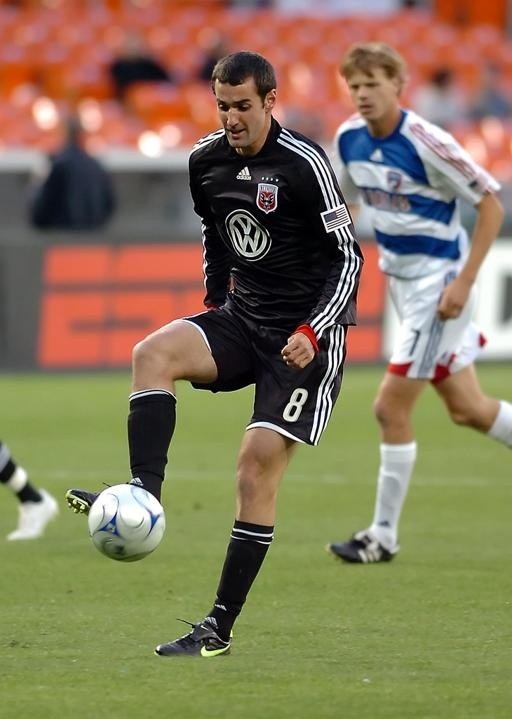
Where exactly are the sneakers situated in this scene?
[7,488,59,543]
[153,617,233,660]
[325,529,401,567]
[64,478,162,518]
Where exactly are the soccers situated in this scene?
[87,482,164,561]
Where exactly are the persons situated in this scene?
[67,52,365,658]
[0,1,511,178]
[31,119,116,232]
[325,43,512,567]
[0,439,58,542]
